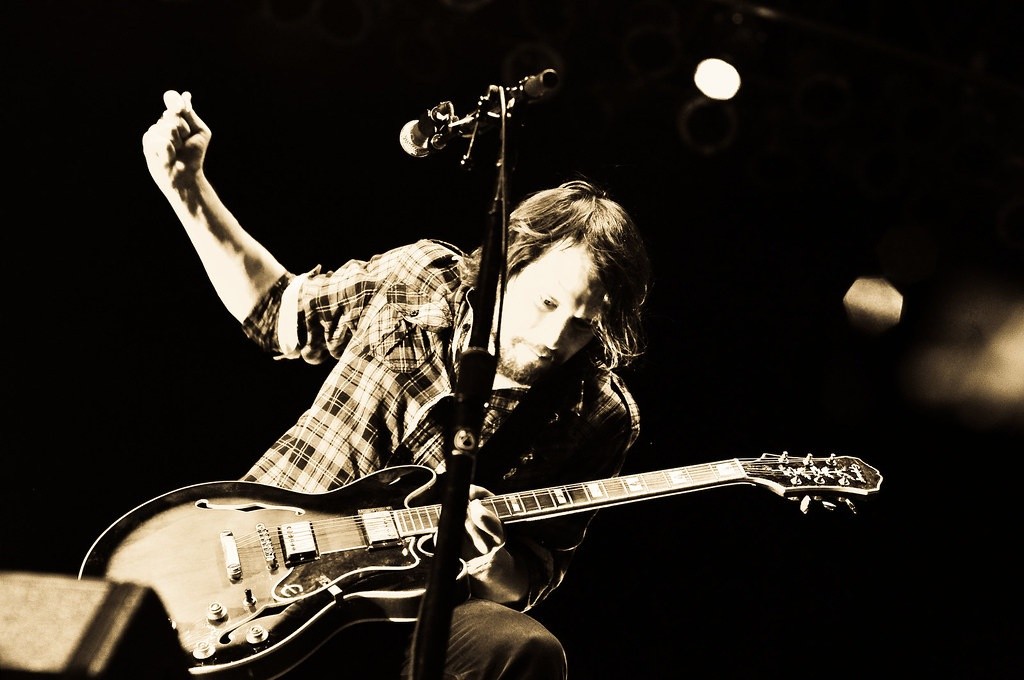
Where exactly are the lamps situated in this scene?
[693,26,769,102]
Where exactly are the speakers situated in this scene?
[0,568,192,680]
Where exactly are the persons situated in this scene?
[142,90,650,680]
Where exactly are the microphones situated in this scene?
[398,101,449,159]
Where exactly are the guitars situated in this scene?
[76,449,890,680]
